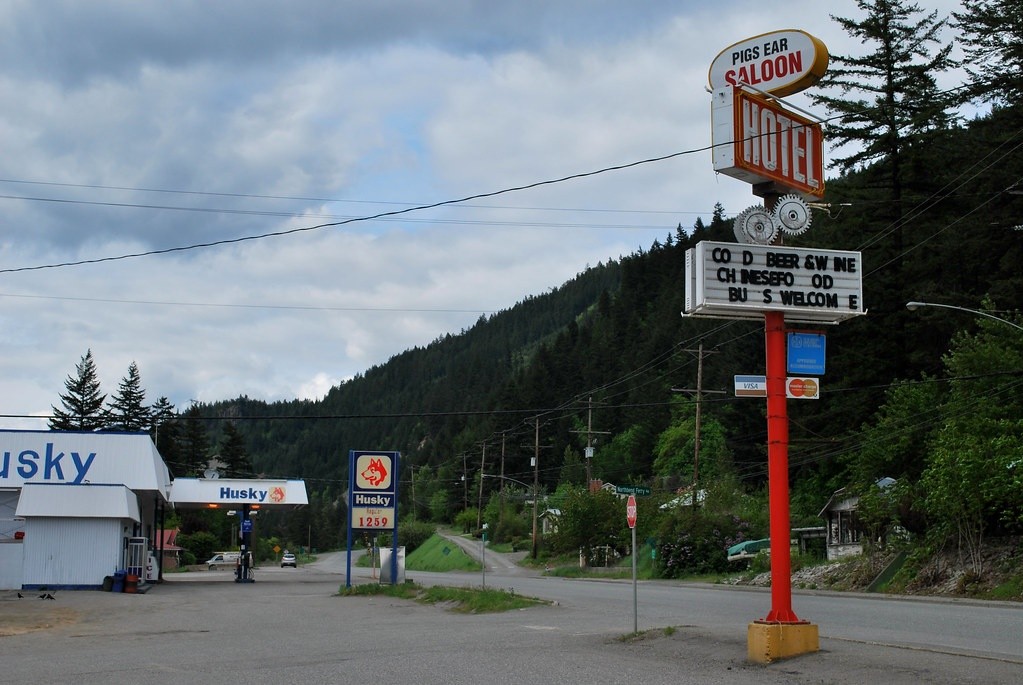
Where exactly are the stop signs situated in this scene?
[626,495,636,528]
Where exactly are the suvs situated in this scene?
[281,554,296,568]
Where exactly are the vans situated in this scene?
[206,551,253,568]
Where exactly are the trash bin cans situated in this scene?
[113,570,127,591]
[125,574,138,593]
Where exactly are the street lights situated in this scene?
[481,473,538,559]
